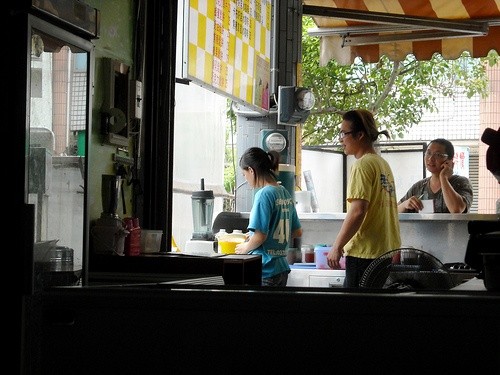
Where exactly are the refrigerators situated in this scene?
[0,0,102,285]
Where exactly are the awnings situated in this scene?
[303,0,500,66]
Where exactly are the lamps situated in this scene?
[277,85,315,126]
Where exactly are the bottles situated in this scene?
[215,228,246,256]
[287,244,316,265]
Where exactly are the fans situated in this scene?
[358,247,454,292]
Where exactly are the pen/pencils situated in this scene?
[418,194,424,200]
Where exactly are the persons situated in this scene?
[397,139,473,214]
[326,111,401,287]
[235,147,302,287]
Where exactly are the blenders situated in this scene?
[185,190,217,255]
[91,175,131,256]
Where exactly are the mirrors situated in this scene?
[25,15,95,286]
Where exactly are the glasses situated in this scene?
[424,151,449,160]
[337,130,352,140]
